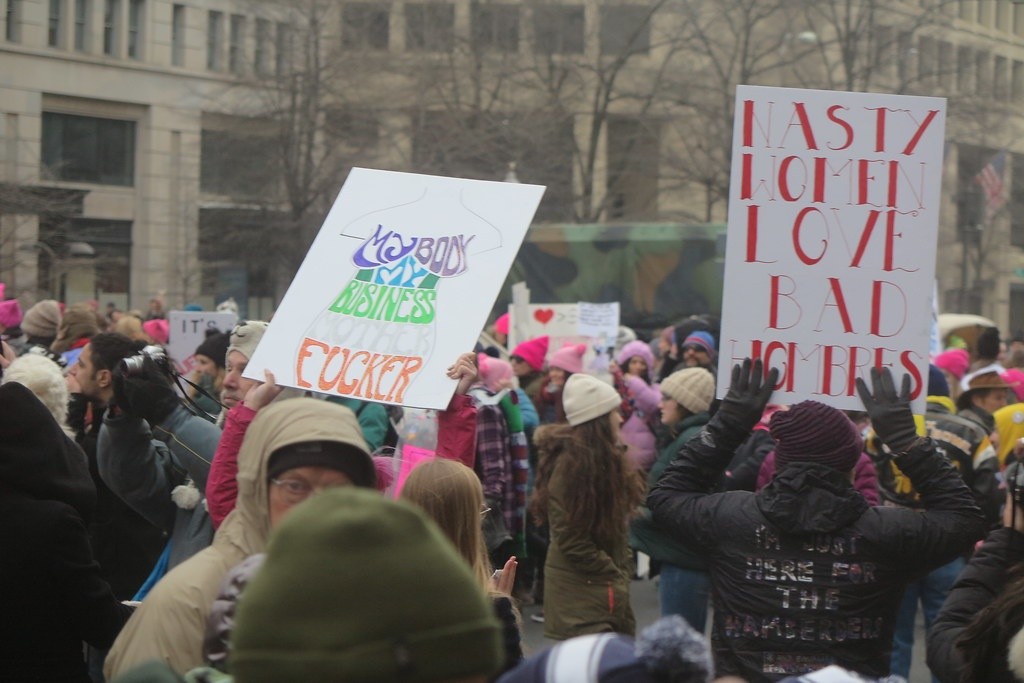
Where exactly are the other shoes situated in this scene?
[531,612,545,622]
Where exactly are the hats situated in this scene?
[0,290,269,372]
[229,486,503,683]
[618,341,654,375]
[267,441,375,488]
[681,330,715,359]
[549,342,587,373]
[661,326,673,345]
[659,366,715,412]
[928,326,1024,500]
[769,400,864,472]
[633,614,715,683]
[495,312,509,335]
[563,373,621,426]
[512,336,549,370]
[616,326,636,349]
[478,352,512,394]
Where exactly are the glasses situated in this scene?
[683,343,705,353]
[509,355,523,363]
[270,477,357,504]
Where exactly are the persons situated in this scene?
[534,314,1023,683]
[0,296,544,683]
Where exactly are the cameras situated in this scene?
[120,346,167,379]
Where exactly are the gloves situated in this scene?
[110,347,176,421]
[708,356,780,446]
[854,367,920,448]
